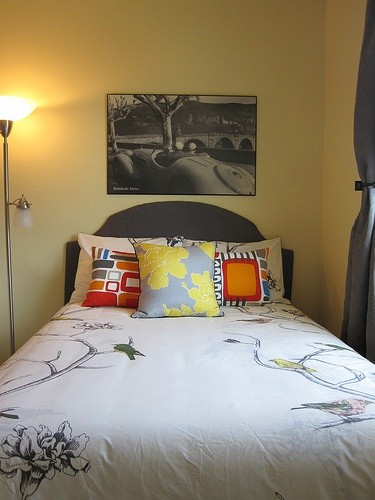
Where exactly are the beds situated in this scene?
[0,200,374,500]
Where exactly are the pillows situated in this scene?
[130,241,224,319]
[71,232,183,307]
[183,237,292,306]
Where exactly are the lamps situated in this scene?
[8,194,34,229]
[0,94,38,357]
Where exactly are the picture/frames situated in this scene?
[106,93,257,197]
